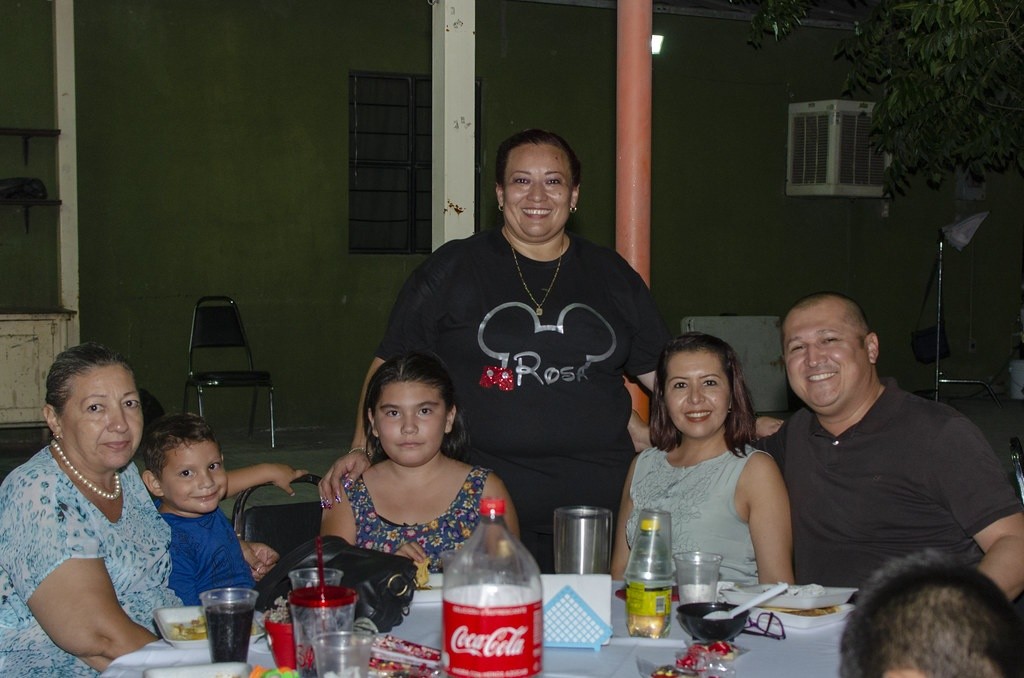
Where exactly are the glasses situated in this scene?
[740,612,787,641]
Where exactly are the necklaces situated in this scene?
[501,227,566,318]
[50,439,121,500]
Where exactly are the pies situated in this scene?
[176,615,256,639]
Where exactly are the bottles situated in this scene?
[440,497,544,677]
[624,519,675,639]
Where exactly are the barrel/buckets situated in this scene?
[1008,360,1024,399]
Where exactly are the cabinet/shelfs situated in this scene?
[0,307,78,447]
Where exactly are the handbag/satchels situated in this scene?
[250,535,419,634]
[910,324,950,365]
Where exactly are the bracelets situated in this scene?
[348,446,373,459]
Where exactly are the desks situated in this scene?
[93,574,856,678]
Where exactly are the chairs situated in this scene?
[231,474,324,562]
[183,296,275,449]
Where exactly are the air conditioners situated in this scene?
[786,100,894,195]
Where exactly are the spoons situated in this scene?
[703,583,789,619]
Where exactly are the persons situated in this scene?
[0,347,282,678]
[139,411,309,598]
[319,347,521,573]
[838,548,1024,678]
[317,129,786,573]
[627,291,1024,604]
[610,330,795,587]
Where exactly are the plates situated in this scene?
[718,582,860,631]
[411,573,443,604]
[152,606,265,650]
[142,661,253,678]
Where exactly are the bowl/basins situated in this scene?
[676,602,752,642]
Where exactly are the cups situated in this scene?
[622,508,678,585]
[199,588,259,664]
[672,552,723,605]
[310,631,374,678]
[289,567,343,590]
[288,587,359,678]
[553,506,612,575]
[263,613,297,670]
